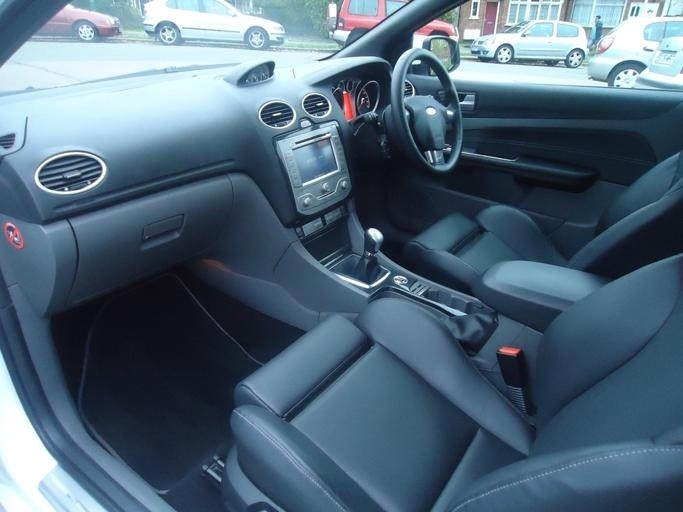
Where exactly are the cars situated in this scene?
[583,14,683,84]
[29,0,119,43]
[471,18,588,67]
[140,0,287,54]
[632,37,683,86]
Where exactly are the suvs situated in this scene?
[326,0,460,58]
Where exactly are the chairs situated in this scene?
[220,253,683,511]
[402,152,682,290]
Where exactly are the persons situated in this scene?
[588,16,603,49]
